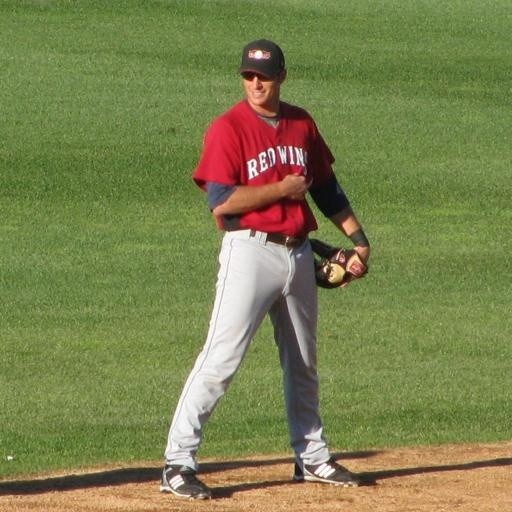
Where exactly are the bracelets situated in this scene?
[349,228,370,248]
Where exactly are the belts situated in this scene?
[250,229,305,249]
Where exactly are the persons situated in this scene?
[160,38,372,500]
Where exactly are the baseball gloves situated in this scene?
[310,238,368,289]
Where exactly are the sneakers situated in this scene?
[159,464,215,500]
[292,456,359,485]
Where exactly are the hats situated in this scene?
[240,39,284,80]
